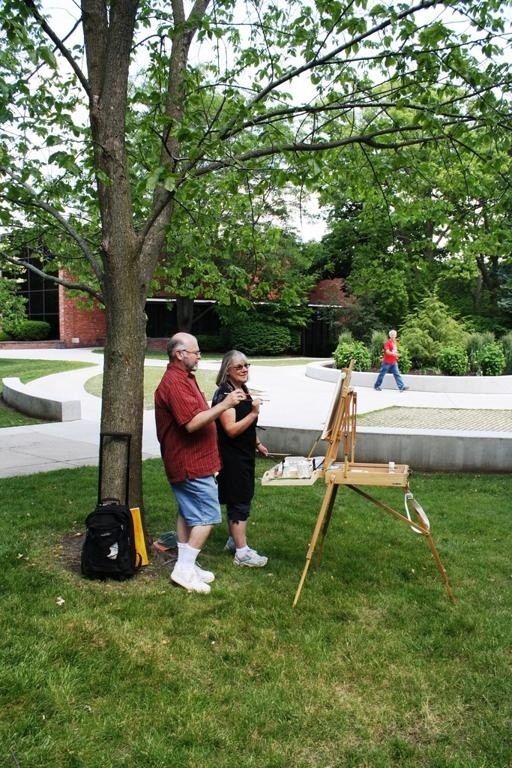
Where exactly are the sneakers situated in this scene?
[232,549,268,568]
[399,387,408,392]
[196,567,215,583]
[171,567,211,595]
[375,384,381,391]
[225,536,258,555]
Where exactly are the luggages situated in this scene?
[80,432,142,581]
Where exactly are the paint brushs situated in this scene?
[245,399,270,401]
[224,393,253,395]
[268,453,291,456]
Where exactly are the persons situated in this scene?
[153,330,245,596]
[210,349,269,568]
[373,329,409,392]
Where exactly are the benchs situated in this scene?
[1,376,81,422]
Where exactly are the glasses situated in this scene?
[184,350,201,356]
[231,364,250,370]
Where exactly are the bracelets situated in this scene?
[255,442,261,447]
